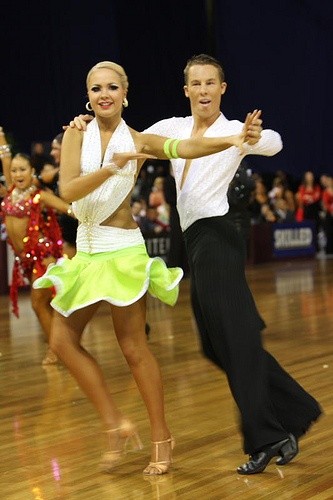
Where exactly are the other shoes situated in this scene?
[41,341,59,366]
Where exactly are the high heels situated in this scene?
[143,434,176,476]
[98,418,144,473]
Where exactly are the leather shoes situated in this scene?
[237,437,292,475]
[276,433,299,465]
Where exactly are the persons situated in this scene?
[63,53,300,475]
[1,125,78,363]
[40,132,63,188]
[131,175,171,238]
[50,60,262,477]
[255,171,333,255]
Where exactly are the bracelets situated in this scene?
[106,160,134,178]
[2,143,11,158]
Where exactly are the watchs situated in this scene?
[67,204,74,217]
